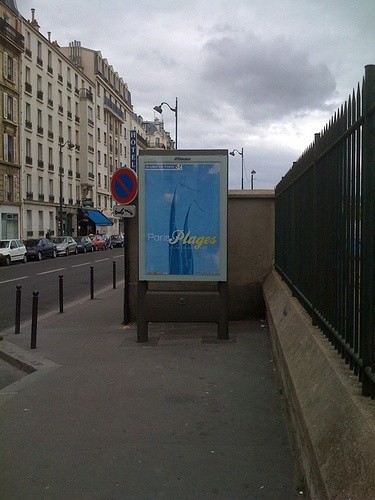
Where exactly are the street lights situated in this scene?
[229,148,244,190]
[251,170,256,190]
[58,140,75,235]
[153,97,178,149]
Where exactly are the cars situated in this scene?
[0,238,28,266]
[89,235,106,251]
[72,236,94,253]
[24,238,58,261]
[51,236,78,256]
[98,233,125,249]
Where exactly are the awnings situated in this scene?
[82,209,114,226]
